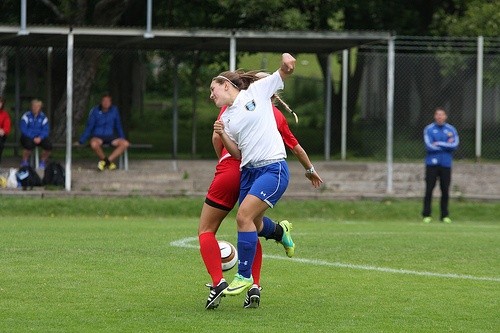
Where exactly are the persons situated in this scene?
[422,108,459,223]
[72,96,130,171]
[20,99,52,169]
[209,53,297,297]
[0,96,11,164]
[196,73,325,308]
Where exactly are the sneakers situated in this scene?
[275,220,295,258]
[220,273,254,296]
[204,278,229,310]
[244,284,263,309]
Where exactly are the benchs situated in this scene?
[2,142,154,170]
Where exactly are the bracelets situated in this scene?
[307,166,315,173]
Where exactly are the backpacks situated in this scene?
[16,166,41,190]
[42,163,66,185]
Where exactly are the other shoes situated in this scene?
[442,217,451,224]
[108,162,116,170]
[423,216,432,223]
[97,160,106,171]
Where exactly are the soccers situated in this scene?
[216,240,239,272]
